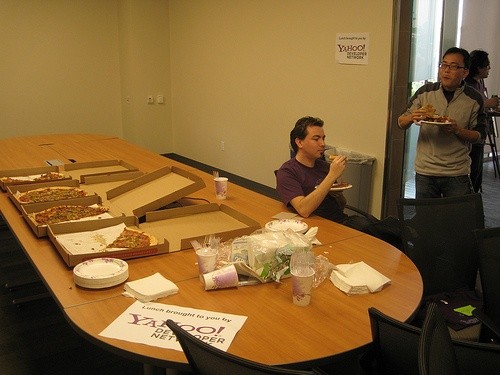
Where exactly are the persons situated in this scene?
[399,46,500,300]
[277,116,406,251]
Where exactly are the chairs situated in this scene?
[0,212,137,375]
[167,170,500,375]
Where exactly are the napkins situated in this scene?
[123,260,389,303]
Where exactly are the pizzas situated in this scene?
[106,227,150,249]
[329,154,349,160]
[16,186,87,203]
[418,104,436,118]
[332,182,349,188]
[32,205,110,225]
[1,172,64,183]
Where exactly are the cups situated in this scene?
[290,268,315,306]
[196,248,218,276]
[203,263,239,290]
[213,177,228,199]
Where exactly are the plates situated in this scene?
[420,120,453,125]
[314,183,352,191]
[73,257,129,289]
[264,218,308,234]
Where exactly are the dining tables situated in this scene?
[0,133,425,375]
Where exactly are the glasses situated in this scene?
[439,63,467,69]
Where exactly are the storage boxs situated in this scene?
[0,159,261,267]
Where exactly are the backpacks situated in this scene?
[361,217,432,287]
